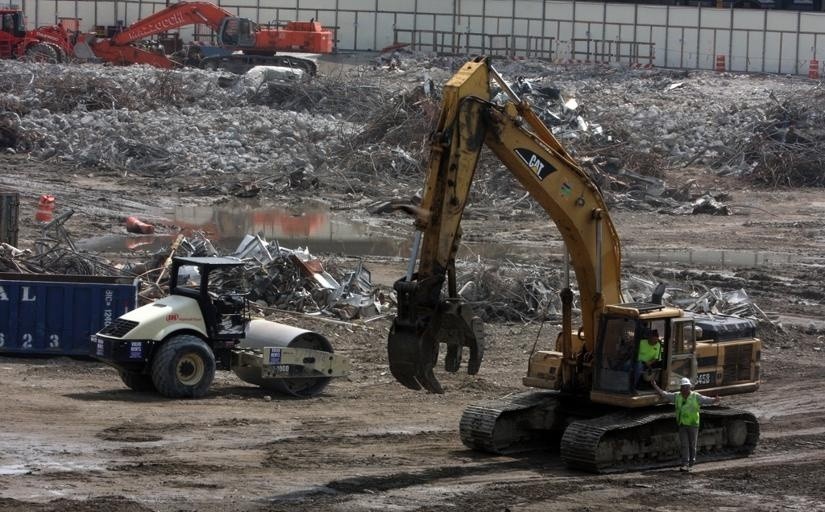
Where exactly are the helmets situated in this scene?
[679,377,691,385]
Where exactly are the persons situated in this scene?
[646,374,722,472]
[622,328,663,389]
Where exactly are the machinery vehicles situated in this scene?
[94,255,350,400]
[386,52,764,476]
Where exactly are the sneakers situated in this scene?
[680,466,689,472]
